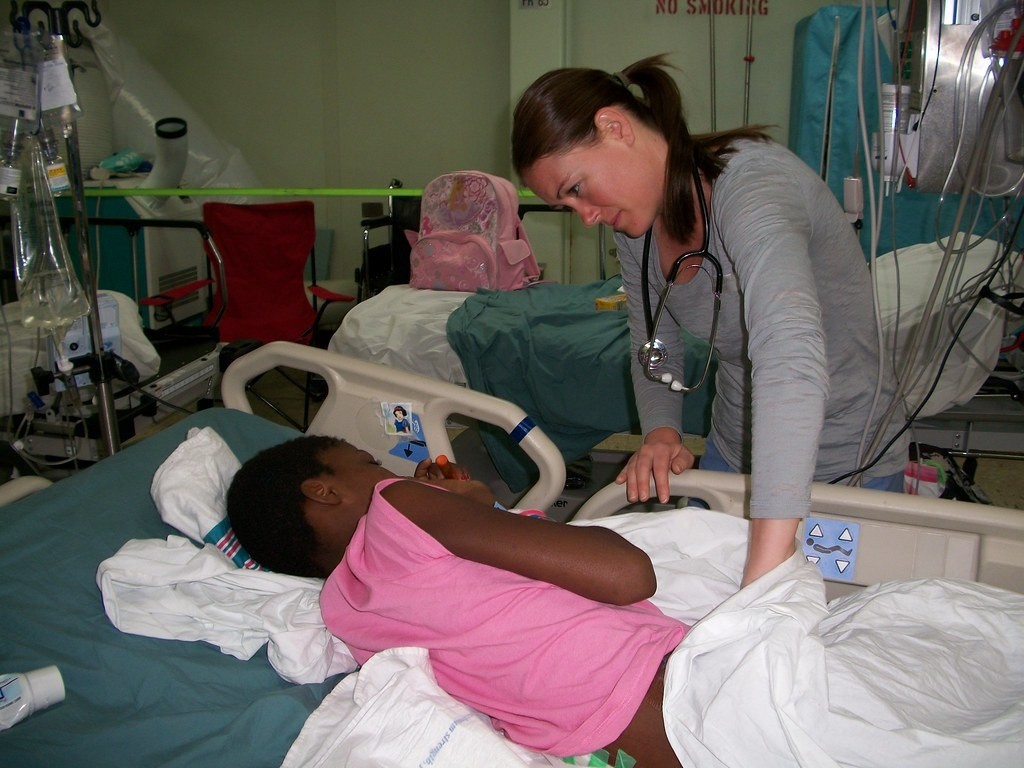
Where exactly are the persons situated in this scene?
[513,54,910,591]
[225,436,685,767]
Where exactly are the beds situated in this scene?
[326,232,1024,525]
[0,407,1024,768]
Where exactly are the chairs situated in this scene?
[200,201,357,432]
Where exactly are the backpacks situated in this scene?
[404,170,541,292]
